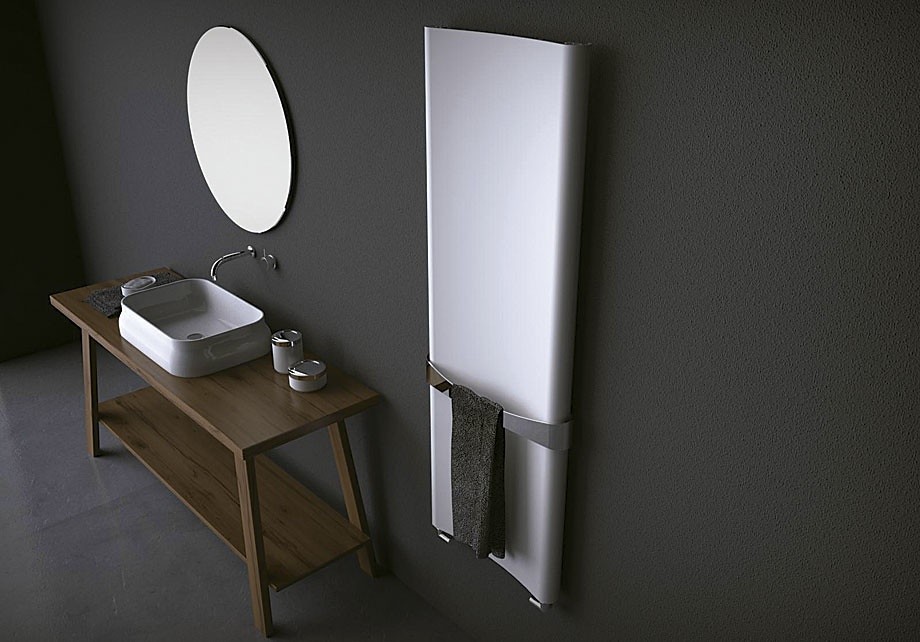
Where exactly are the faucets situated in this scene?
[209,246,258,282]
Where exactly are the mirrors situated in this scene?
[186,27,294,235]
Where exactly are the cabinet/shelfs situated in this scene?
[48,264,384,639]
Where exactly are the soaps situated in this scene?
[125,277,152,290]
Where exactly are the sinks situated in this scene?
[118,278,272,377]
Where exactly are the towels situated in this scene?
[448,383,508,561]
[85,270,173,319]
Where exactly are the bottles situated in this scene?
[272,328,329,392]
